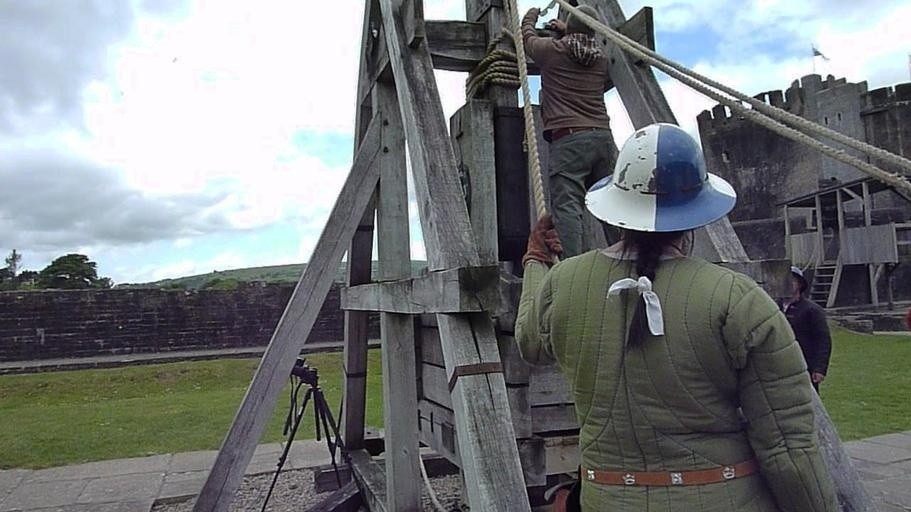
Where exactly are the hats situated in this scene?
[791,265,808,291]
[566,4,600,32]
[583,116,738,234]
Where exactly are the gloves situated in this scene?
[521,213,563,268]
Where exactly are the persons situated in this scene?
[514,123,844,512]
[524,2,620,264]
[777,266,834,396]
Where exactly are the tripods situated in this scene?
[261,384,372,512]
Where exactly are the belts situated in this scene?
[552,126,589,139]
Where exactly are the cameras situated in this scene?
[291,358,318,384]
